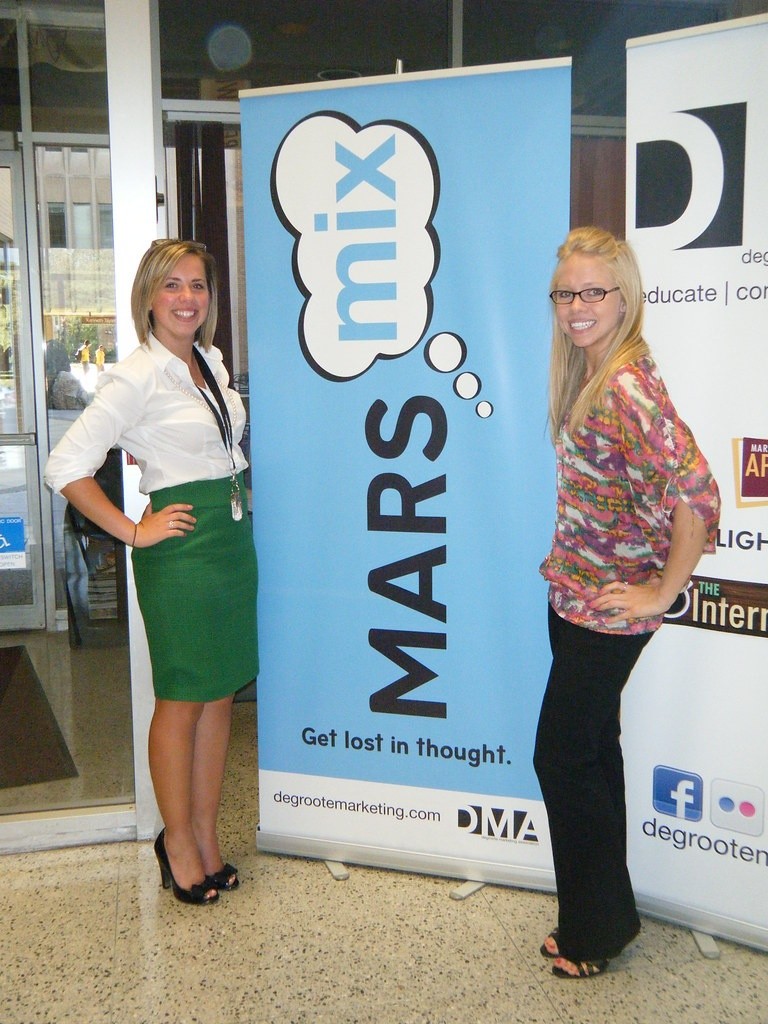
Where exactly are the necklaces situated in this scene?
[160,366,236,427]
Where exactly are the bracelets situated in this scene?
[132,525,137,546]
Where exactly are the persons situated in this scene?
[80,340,92,373]
[45,340,88,409]
[533,227,718,981]
[43,240,260,902]
[96,346,104,371]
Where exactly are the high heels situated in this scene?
[154,827,219,905]
[204,862,239,891]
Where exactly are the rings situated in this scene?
[169,522,174,528]
[624,584,626,591]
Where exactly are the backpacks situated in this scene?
[75,346,87,360]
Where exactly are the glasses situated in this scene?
[549,286,623,305]
[152,239,206,251]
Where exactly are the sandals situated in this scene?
[540,928,568,958]
[552,948,612,979]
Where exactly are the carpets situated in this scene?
[0,645,78,790]
